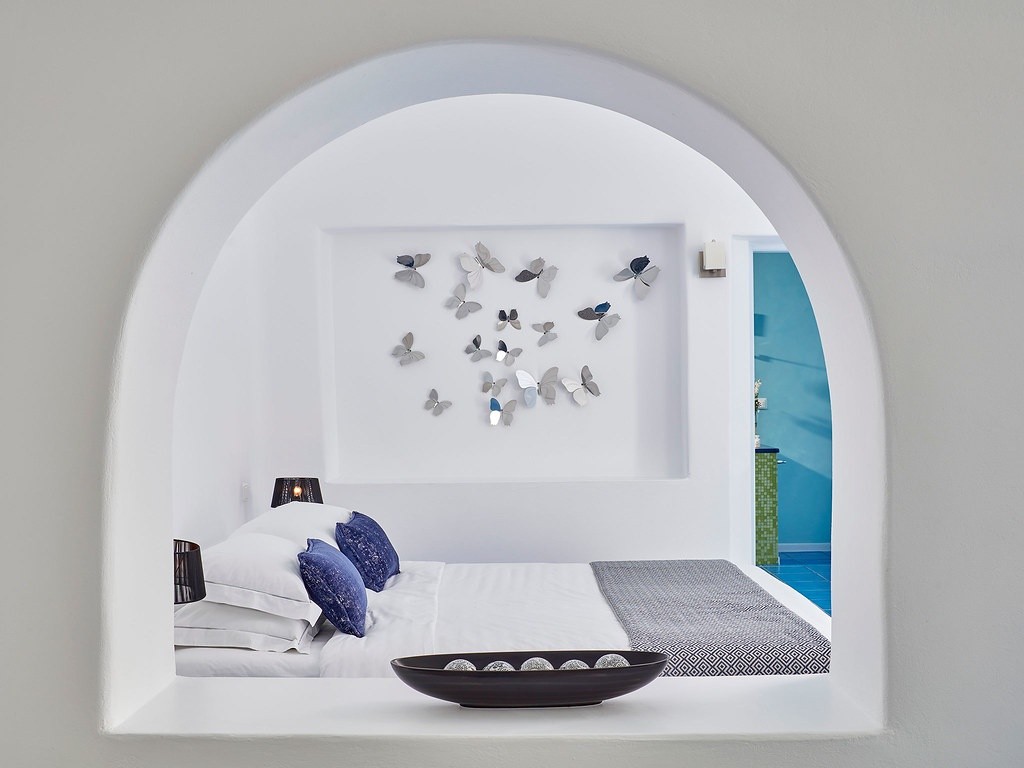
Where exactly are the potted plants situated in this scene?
[755,378,762,448]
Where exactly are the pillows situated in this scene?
[200,533,323,626]
[335,510,402,593]
[174,597,328,654]
[231,501,353,552]
[297,538,367,638]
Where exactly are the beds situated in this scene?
[176,562,831,677]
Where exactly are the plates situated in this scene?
[390,649,668,710]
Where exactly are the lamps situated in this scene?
[174,538,207,605]
[270,476,323,508]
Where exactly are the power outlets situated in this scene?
[758,398,768,410]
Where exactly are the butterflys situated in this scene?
[424,388,452,416]
[613,254,661,299]
[391,332,426,367]
[444,240,601,427]
[393,253,432,289]
[578,301,622,342]
